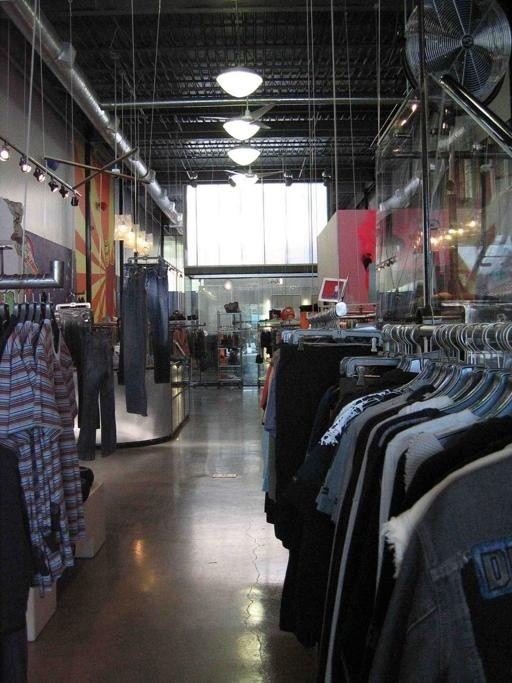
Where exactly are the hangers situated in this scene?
[256,304,512,424]
[0,253,202,344]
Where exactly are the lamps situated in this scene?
[215,0,263,187]
[0,136,82,206]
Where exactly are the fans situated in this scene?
[400,0,511,160]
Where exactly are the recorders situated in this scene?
[54,302,93,325]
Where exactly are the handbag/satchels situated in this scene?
[300,303,318,311]
[188,314,197,319]
[224,301,238,312]
[269,309,281,319]
[169,309,186,320]
